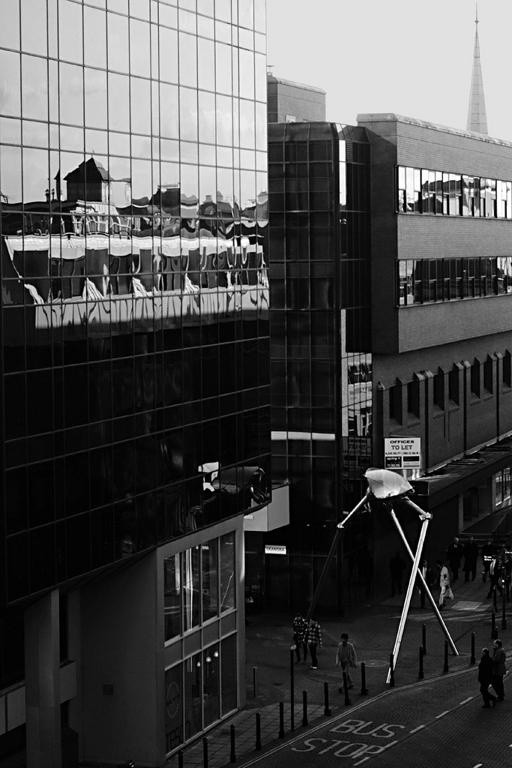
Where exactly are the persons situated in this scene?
[307,617,322,670]
[481,537,497,575]
[415,560,432,608]
[438,563,454,611]
[478,648,497,708]
[447,536,464,580]
[462,536,478,582]
[336,633,358,694]
[491,639,506,702]
[293,613,308,665]
[389,552,406,598]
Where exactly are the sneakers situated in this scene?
[480,694,505,710]
[419,576,510,611]
[293,658,354,695]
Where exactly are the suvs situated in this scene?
[211,467,266,508]
